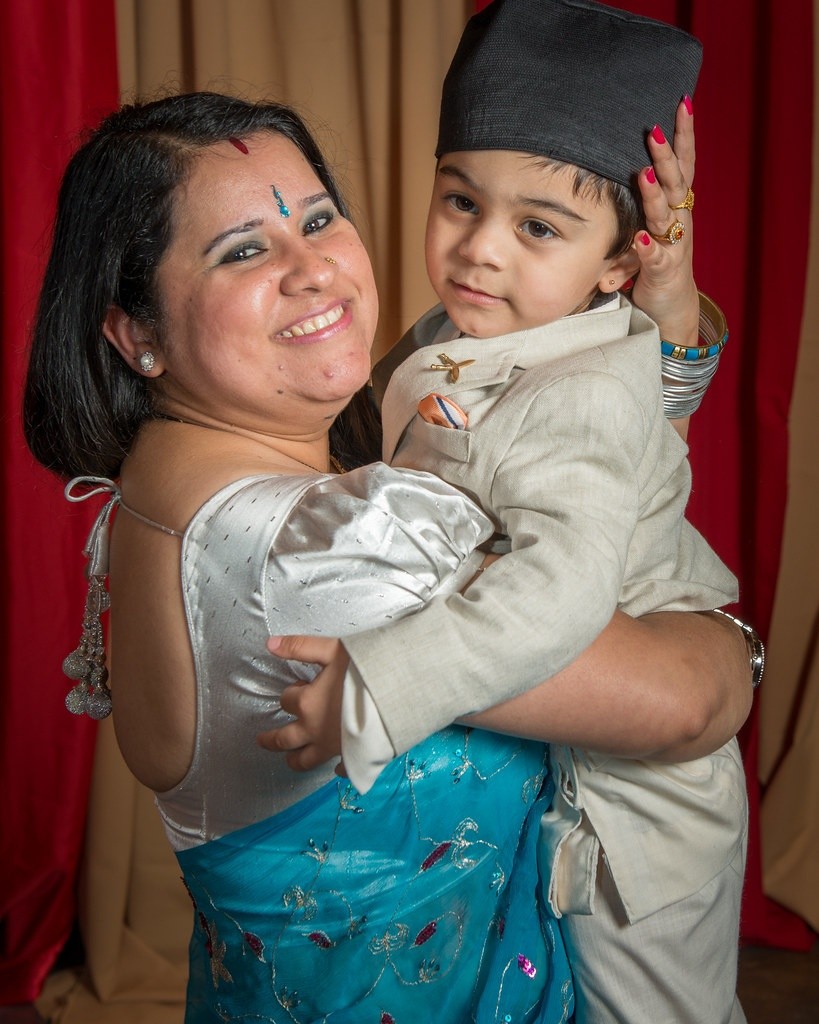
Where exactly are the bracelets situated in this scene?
[714,605,764,687]
[627,283,728,418]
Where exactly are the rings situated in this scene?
[668,186,695,211]
[653,219,685,244]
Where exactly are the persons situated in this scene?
[19,89,764,1024]
[257,147,751,1024]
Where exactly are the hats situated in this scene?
[435,0,704,192]
